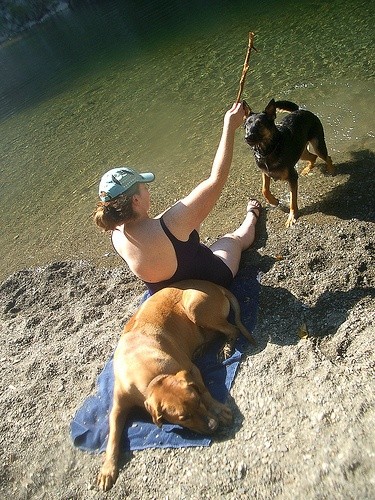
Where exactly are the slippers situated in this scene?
[247,199,261,219]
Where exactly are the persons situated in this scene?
[94,103,266,294]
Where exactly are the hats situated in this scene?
[99,167,155,202]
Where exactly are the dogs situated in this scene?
[93,279,256,495]
[240,99,332,229]
